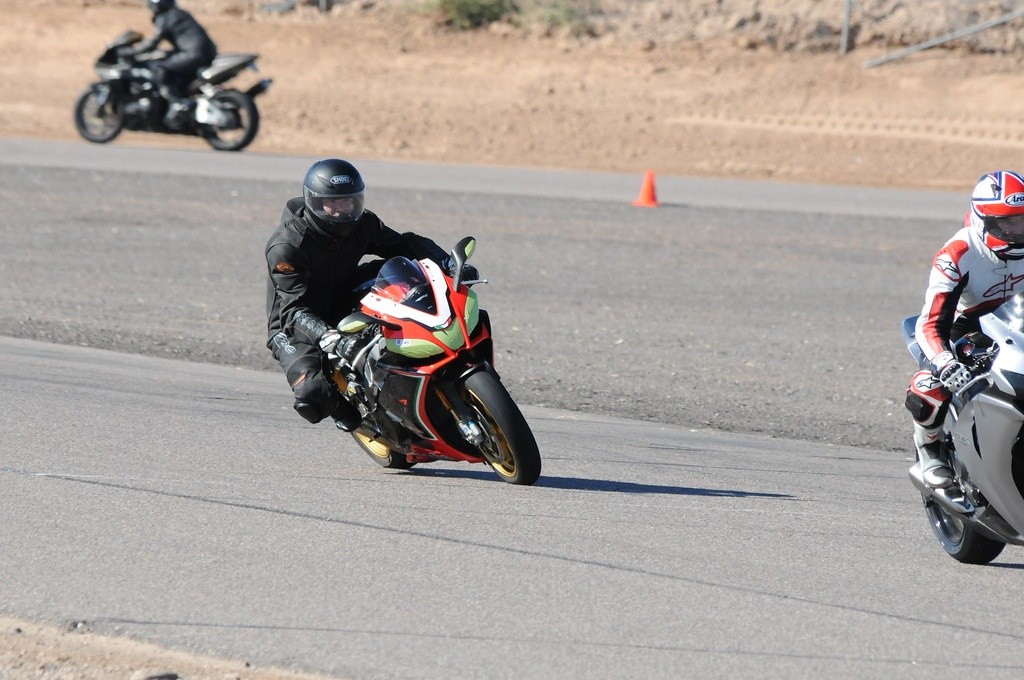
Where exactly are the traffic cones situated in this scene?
[632,167,661,209]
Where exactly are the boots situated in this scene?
[912,421,954,489]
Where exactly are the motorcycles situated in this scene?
[73,28,273,152]
[328,236,542,485]
[901,292,1024,564]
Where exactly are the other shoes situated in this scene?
[164,97,196,123]
[331,403,362,432]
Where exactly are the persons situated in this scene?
[265,158,480,433]
[914,169,1023,488]
[113,0,217,124]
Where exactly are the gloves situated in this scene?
[448,263,479,288]
[929,350,973,393]
[332,334,366,363]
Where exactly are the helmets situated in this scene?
[302,159,367,249]
[149,0,175,13]
[970,170,1024,262]
